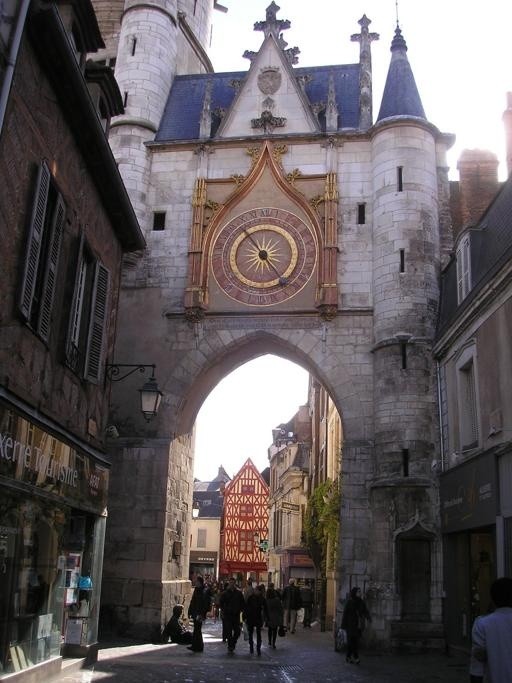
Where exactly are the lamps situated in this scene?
[103,358,164,423]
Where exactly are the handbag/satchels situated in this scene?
[334,628,349,653]
[278,624,288,637]
[243,619,249,641]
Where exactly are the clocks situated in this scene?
[211,207,317,307]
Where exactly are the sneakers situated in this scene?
[346,657,361,665]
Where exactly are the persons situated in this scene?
[341,587,372,664]
[161,573,314,656]
[470,576,512,683]
